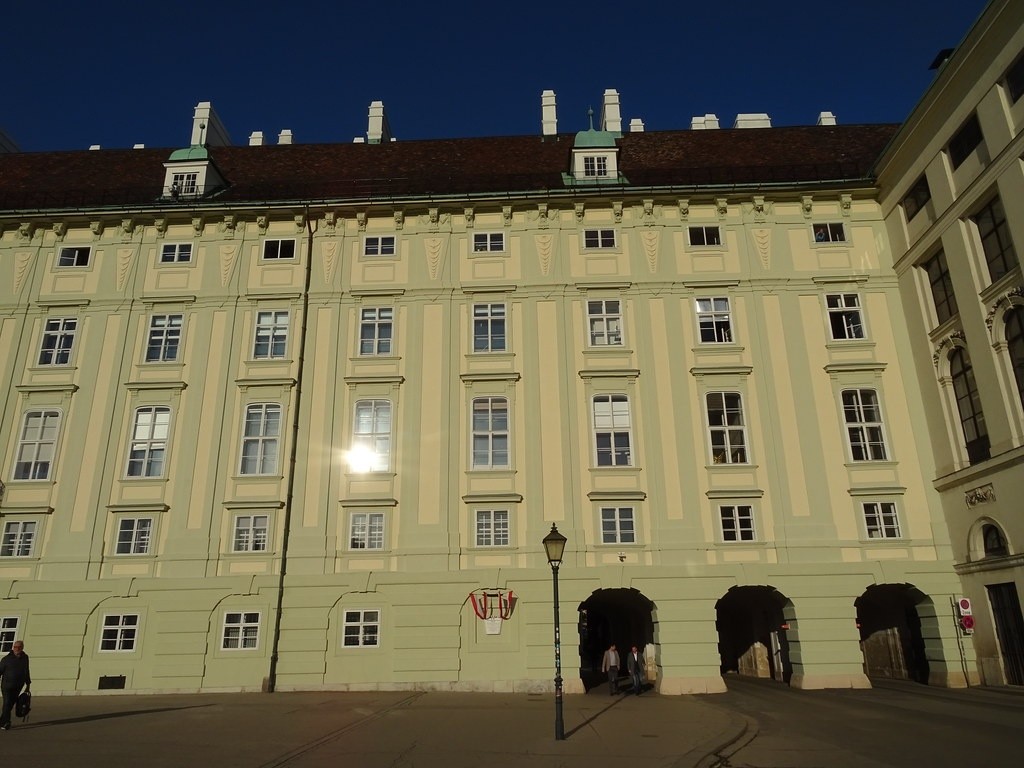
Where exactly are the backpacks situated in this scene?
[16,684,32,723]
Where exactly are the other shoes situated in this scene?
[1,722,11,731]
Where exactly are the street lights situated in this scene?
[542,521,568,740]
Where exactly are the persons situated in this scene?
[627,645,646,697]
[601,643,621,696]
[0,641,32,730]
